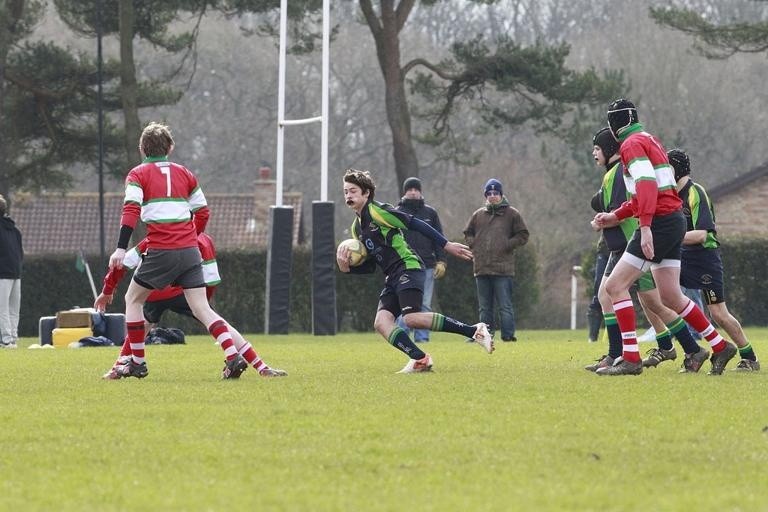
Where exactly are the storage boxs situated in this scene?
[52,327,93,347]
[55,310,92,329]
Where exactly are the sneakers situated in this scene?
[0,339,18,349]
[465,321,495,355]
[709,342,737,375]
[260,368,289,378]
[101,354,150,380]
[394,352,434,374]
[220,352,248,380]
[678,346,710,375]
[584,355,615,373]
[595,358,644,375]
[642,346,677,369]
[731,356,761,372]
[501,335,518,342]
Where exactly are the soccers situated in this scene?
[337,239,367,267]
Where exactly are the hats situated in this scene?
[402,177,422,195]
[483,178,503,199]
[593,127,620,167]
[607,99,639,143]
[667,149,691,184]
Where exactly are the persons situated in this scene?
[588,233,610,342]
[392,177,449,343]
[108,126,249,380]
[335,167,496,374]
[0,196,25,349]
[643,149,760,374]
[93,233,287,377]
[595,100,736,374]
[585,127,709,375]
[462,179,529,344]
